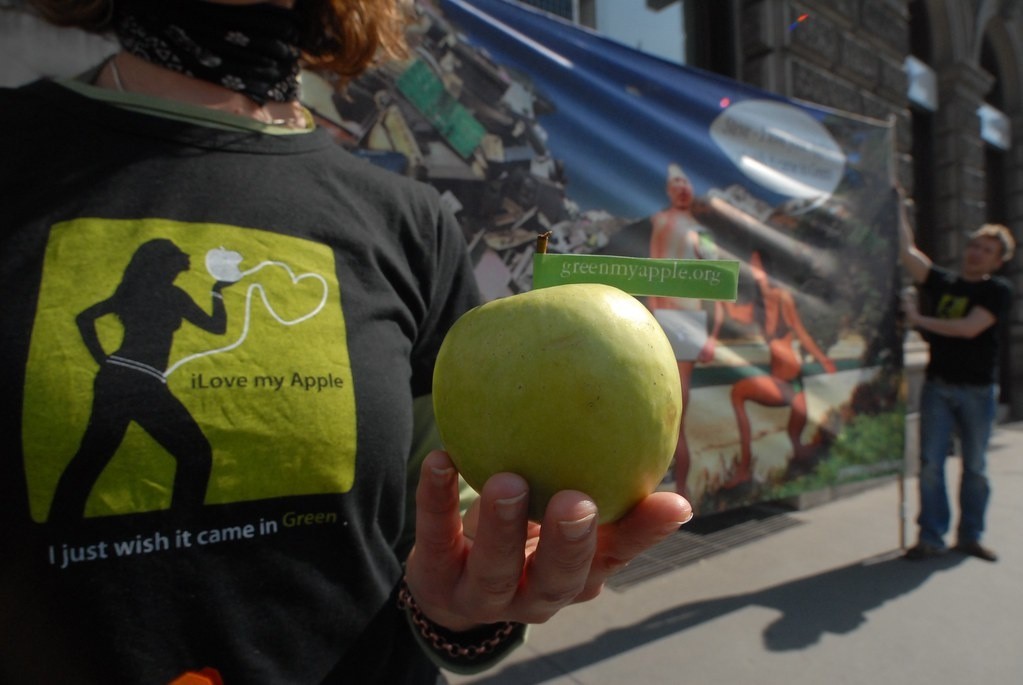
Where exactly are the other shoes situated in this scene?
[957,541,997,562]
[907,542,947,560]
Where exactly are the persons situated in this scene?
[899,186,1015,561]
[645,162,726,505]
[720,248,837,496]
[0,0,694,685]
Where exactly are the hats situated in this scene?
[971,224,1015,262]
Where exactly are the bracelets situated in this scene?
[398,588,516,660]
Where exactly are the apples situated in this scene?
[432,230,682,524]
